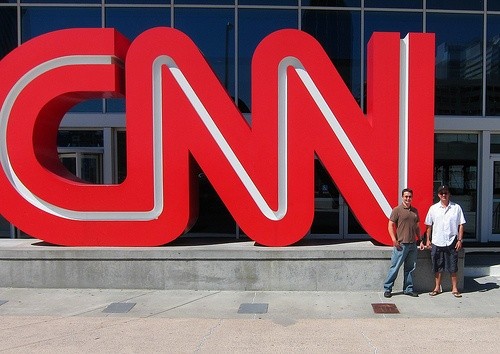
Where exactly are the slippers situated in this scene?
[452,292,462,297]
[429,290,441,296]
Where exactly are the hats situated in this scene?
[438,185,449,193]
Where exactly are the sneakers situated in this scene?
[405,291,418,297]
[384,292,392,297]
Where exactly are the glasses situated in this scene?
[405,196,412,198]
[439,192,449,195]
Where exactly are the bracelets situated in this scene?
[457,240,463,242]
[419,240,423,242]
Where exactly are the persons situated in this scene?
[462,166,477,211]
[425,184,467,298]
[384,189,425,298]
